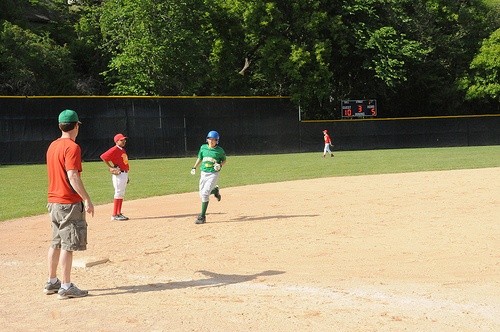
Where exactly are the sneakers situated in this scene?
[43,278,61,294]
[213,185,220,201]
[194,217,208,223]
[112,213,128,220]
[58,281,88,299]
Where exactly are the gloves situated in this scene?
[213,164,221,171]
[191,168,196,175]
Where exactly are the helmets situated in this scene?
[206,131,219,144]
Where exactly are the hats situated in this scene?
[113,133,128,143]
[57,109,82,126]
[323,130,328,132]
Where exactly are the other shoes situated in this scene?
[331,154,334,157]
[323,155,326,157]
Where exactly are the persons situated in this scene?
[321,129,334,158]
[43,109,95,299]
[190,130,226,224]
[100,133,129,220]
